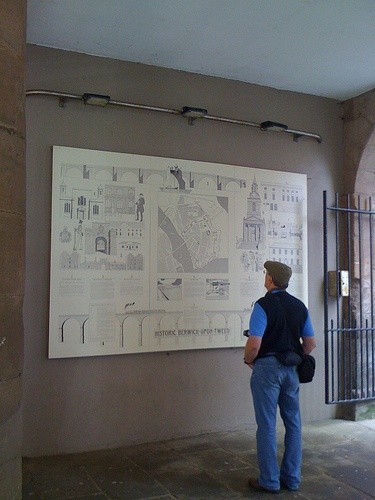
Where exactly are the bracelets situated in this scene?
[244,357,252,364]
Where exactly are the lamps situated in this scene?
[260,121,288,132]
[181,108,207,120]
[81,93,110,107]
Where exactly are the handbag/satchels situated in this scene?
[298,352,315,383]
[275,350,302,366]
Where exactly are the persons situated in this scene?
[244,262,317,494]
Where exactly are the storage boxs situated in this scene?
[328,271,352,297]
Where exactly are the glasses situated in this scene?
[263,270,269,275]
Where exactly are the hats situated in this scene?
[264,261,292,289]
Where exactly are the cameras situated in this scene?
[243,330,250,337]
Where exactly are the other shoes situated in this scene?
[248,477,280,494]
[281,479,298,491]
